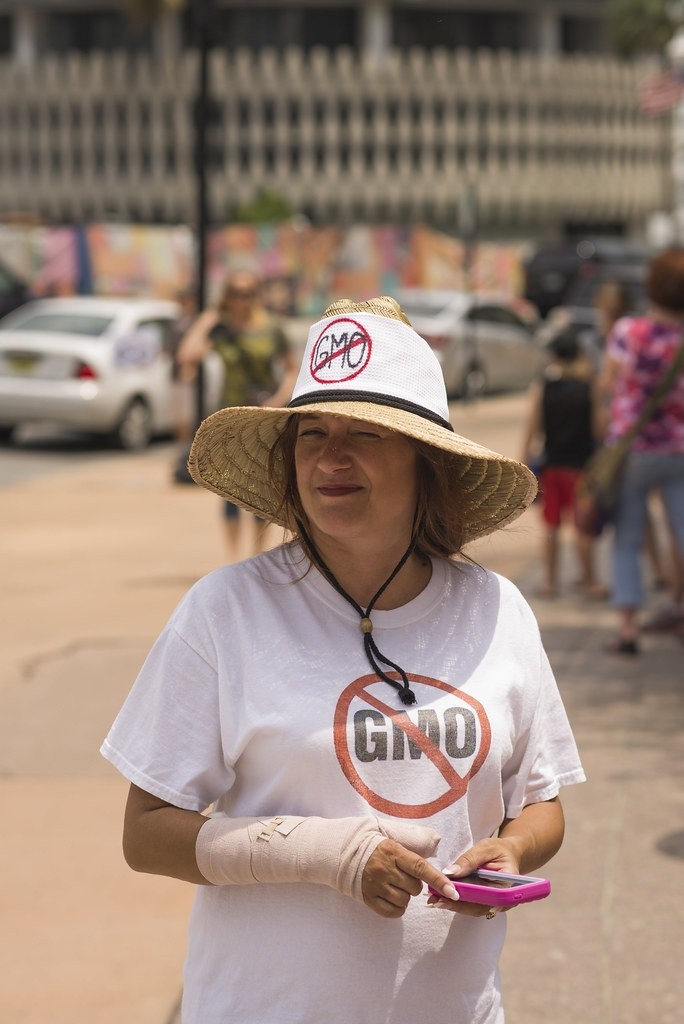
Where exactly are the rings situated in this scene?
[486,906,496,920]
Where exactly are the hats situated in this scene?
[186,296,539,546]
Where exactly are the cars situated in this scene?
[1,293,230,453]
[392,289,548,404]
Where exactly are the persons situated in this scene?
[177,265,302,562]
[100,296,587,1024]
[525,244,684,655]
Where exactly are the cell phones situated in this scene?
[427,868,551,907]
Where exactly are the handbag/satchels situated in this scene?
[574,444,625,529]
[218,324,278,407]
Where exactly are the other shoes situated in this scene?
[635,592,678,628]
[604,630,638,654]
